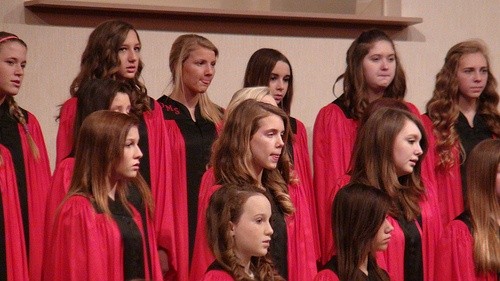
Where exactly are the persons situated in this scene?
[464,137,499,281]
[203,86,280,174]
[348,98,431,281]
[156,34,229,273]
[424,40,500,227]
[41,110,164,281]
[312,184,395,281]
[199,184,287,281]
[0,30,52,280]
[42,74,154,266]
[312,27,433,267]
[187,100,320,281]
[0,145,33,281]
[55,20,163,195]
[242,48,312,207]
